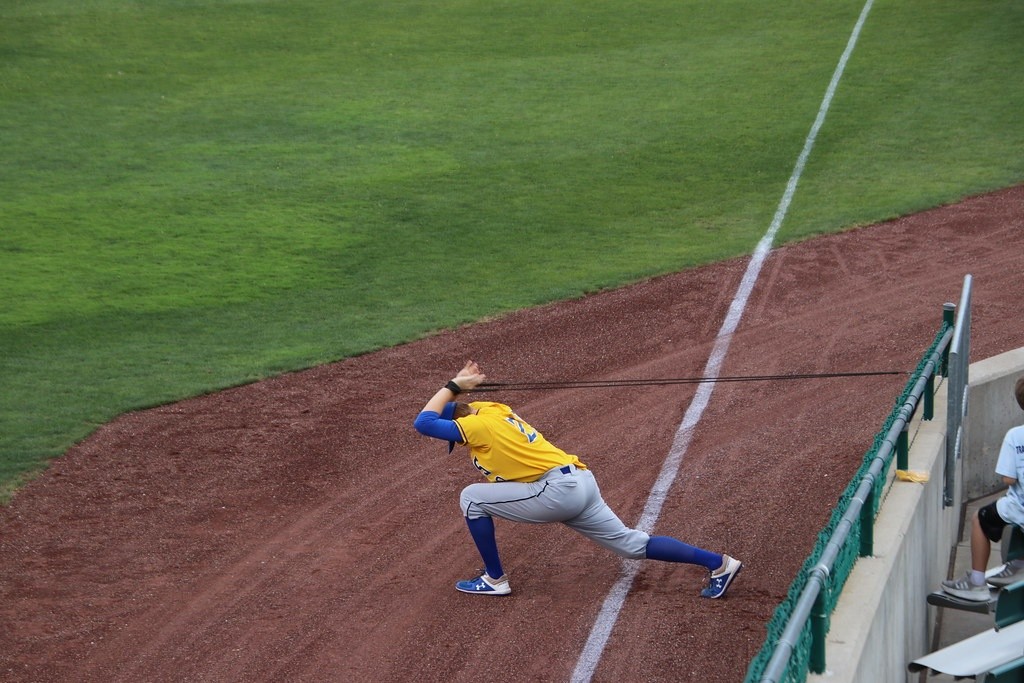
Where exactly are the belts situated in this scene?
[561,465,577,475]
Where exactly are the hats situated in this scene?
[439,401,457,455]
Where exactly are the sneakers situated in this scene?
[940,572,991,601]
[700,553,742,598]
[986,563,1024,586]
[455,572,511,595]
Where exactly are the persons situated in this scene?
[413,360,742,599]
[941,378,1024,601]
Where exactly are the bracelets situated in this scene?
[445,381,461,395]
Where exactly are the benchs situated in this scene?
[906,523,1024,683]
